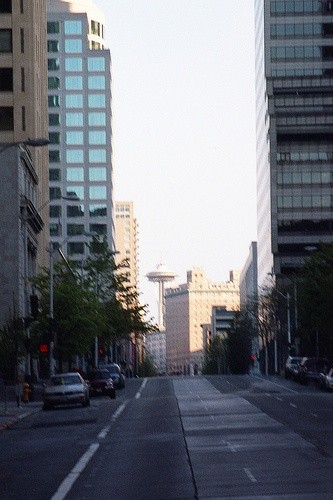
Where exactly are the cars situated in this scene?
[88,368,117,398]
[99,363,127,389]
[41,373,91,410]
[285,356,333,389]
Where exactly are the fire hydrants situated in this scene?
[22,383,31,403]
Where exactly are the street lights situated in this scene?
[48,231,97,379]
[273,288,291,357]
[268,272,300,356]
